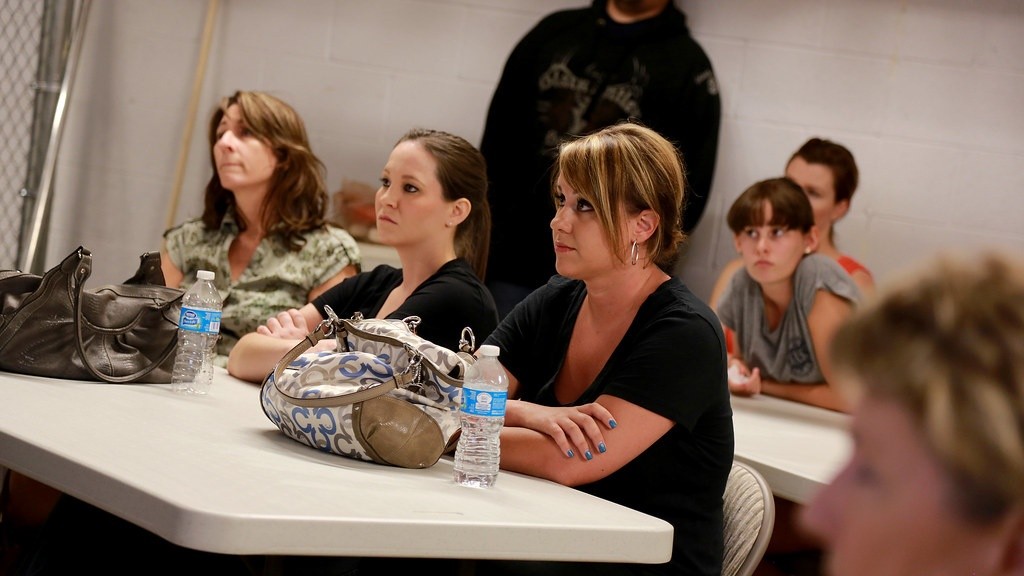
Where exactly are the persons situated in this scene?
[472,124,735,576]
[791,248,1024,576]
[475,0,731,321]
[160,89,363,357]
[226,126,501,383]
[708,137,880,414]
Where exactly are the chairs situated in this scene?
[722,460,776,576]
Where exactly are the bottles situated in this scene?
[169,270,223,396]
[453,345,508,490]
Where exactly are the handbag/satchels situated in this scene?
[259,304,485,468]
[0,246,203,385]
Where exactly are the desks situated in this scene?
[731,391,864,508]
[0,347,676,568]
